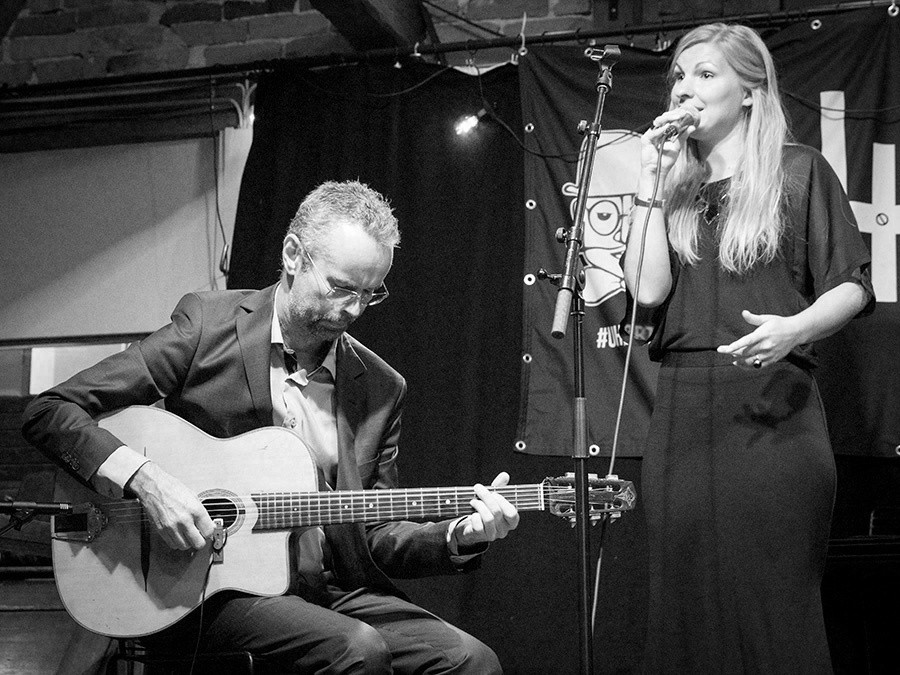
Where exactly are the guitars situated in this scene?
[46,402,639,639]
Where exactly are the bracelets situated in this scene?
[633,196,665,209]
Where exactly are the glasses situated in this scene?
[302,245,389,305]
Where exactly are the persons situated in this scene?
[20,181,520,675]
[620,22,876,674]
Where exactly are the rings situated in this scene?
[754,355,762,369]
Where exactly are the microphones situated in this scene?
[663,105,701,139]
